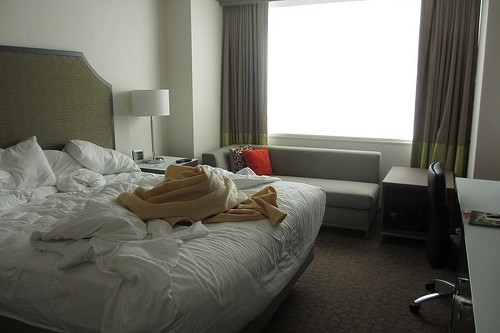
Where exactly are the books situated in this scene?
[468,210,500,228]
[462,209,471,223]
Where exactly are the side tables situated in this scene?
[380,166,455,245]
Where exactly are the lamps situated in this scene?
[131,89,170,164]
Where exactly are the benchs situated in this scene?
[202,144,381,240]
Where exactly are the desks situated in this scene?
[455,176,500,333]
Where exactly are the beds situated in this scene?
[0,45,326,333]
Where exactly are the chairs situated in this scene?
[409,161,472,319]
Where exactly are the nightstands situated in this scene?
[139,155,199,173]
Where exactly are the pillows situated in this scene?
[0,136,141,214]
[229,144,272,175]
